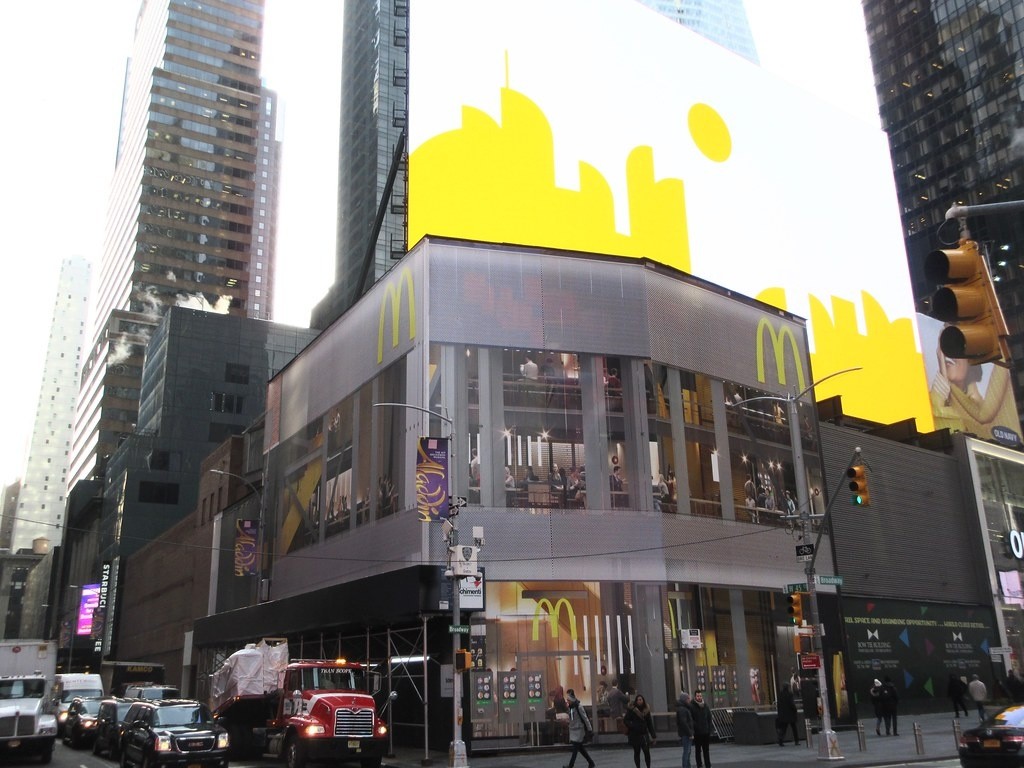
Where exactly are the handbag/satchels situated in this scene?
[584,729,593,744]
[775,717,780,729]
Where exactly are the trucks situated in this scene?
[0,639,60,764]
[52,673,106,730]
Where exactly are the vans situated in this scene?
[123,684,183,702]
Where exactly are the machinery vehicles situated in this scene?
[211,637,399,768]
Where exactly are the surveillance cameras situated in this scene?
[474,572,482,582]
[445,570,454,580]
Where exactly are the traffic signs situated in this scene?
[449,496,467,508]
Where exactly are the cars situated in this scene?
[957,701,1024,768]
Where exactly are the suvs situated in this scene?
[119,698,233,768]
[62,694,118,749]
[93,695,152,761]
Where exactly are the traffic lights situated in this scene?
[787,593,803,627]
[455,649,473,670]
[921,239,1010,367]
[845,464,870,506]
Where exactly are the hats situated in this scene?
[568,694,576,702]
[874,679,882,686]
[680,693,689,703]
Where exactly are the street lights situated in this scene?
[368,401,470,768]
[724,363,866,760]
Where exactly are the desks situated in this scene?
[466,486,524,508]
[579,490,662,510]
[603,712,678,732]
[690,498,785,524]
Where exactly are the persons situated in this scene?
[547,461,586,509]
[562,689,595,768]
[518,465,539,489]
[929,325,983,436]
[753,675,760,704]
[969,674,987,722]
[519,354,814,448]
[676,689,714,768]
[991,678,1011,705]
[609,466,628,508]
[653,472,677,512]
[598,678,630,732]
[471,448,477,486]
[504,466,515,507]
[948,673,968,718]
[379,477,391,518]
[870,678,891,736]
[1006,670,1024,703]
[881,678,899,736]
[546,686,569,719]
[777,682,801,746]
[623,694,657,768]
[745,474,760,524]
[793,673,800,698]
[757,486,795,527]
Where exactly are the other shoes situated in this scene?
[876,728,880,735]
[795,743,800,745]
[894,733,899,736]
[965,711,968,716]
[778,742,784,746]
[955,715,959,718]
[886,732,891,735]
